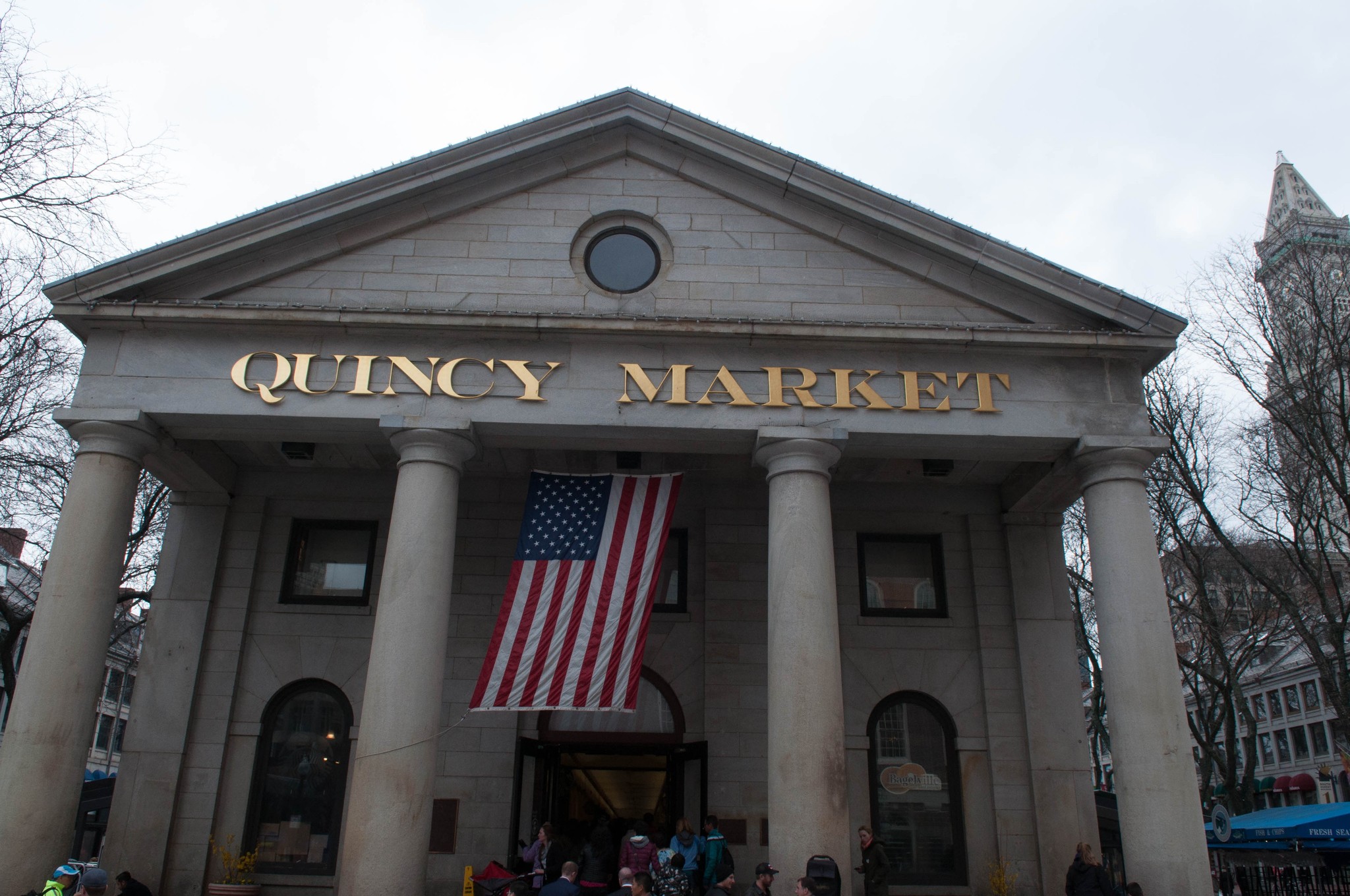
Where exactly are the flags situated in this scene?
[467,471,683,715]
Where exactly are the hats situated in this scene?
[755,863,779,875]
[715,867,735,883]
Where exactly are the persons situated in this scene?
[508,815,735,896]
[77,868,108,896]
[743,862,779,896]
[114,871,153,896]
[794,877,816,896]
[854,825,890,896]
[1066,841,1115,896]
[42,865,81,896]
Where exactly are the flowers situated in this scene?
[208,831,274,885]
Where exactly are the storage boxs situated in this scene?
[256,815,334,866]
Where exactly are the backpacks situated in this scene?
[712,834,735,879]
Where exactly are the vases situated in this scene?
[208,883,263,896]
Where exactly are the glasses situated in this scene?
[507,892,513,896]
[704,823,708,826]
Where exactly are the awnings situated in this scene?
[1198,773,1316,801]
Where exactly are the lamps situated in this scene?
[617,449,643,471]
[921,457,956,477]
[282,441,317,460]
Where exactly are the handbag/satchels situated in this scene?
[640,871,655,887]
